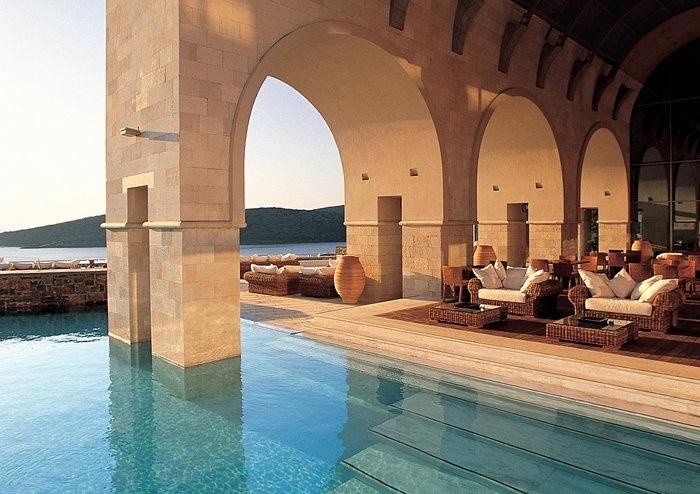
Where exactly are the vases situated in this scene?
[332,255,367,305]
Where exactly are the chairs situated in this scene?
[431,239,700,353]
[1,258,105,270]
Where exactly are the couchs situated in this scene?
[239,253,338,299]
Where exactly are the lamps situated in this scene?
[120,125,140,138]
